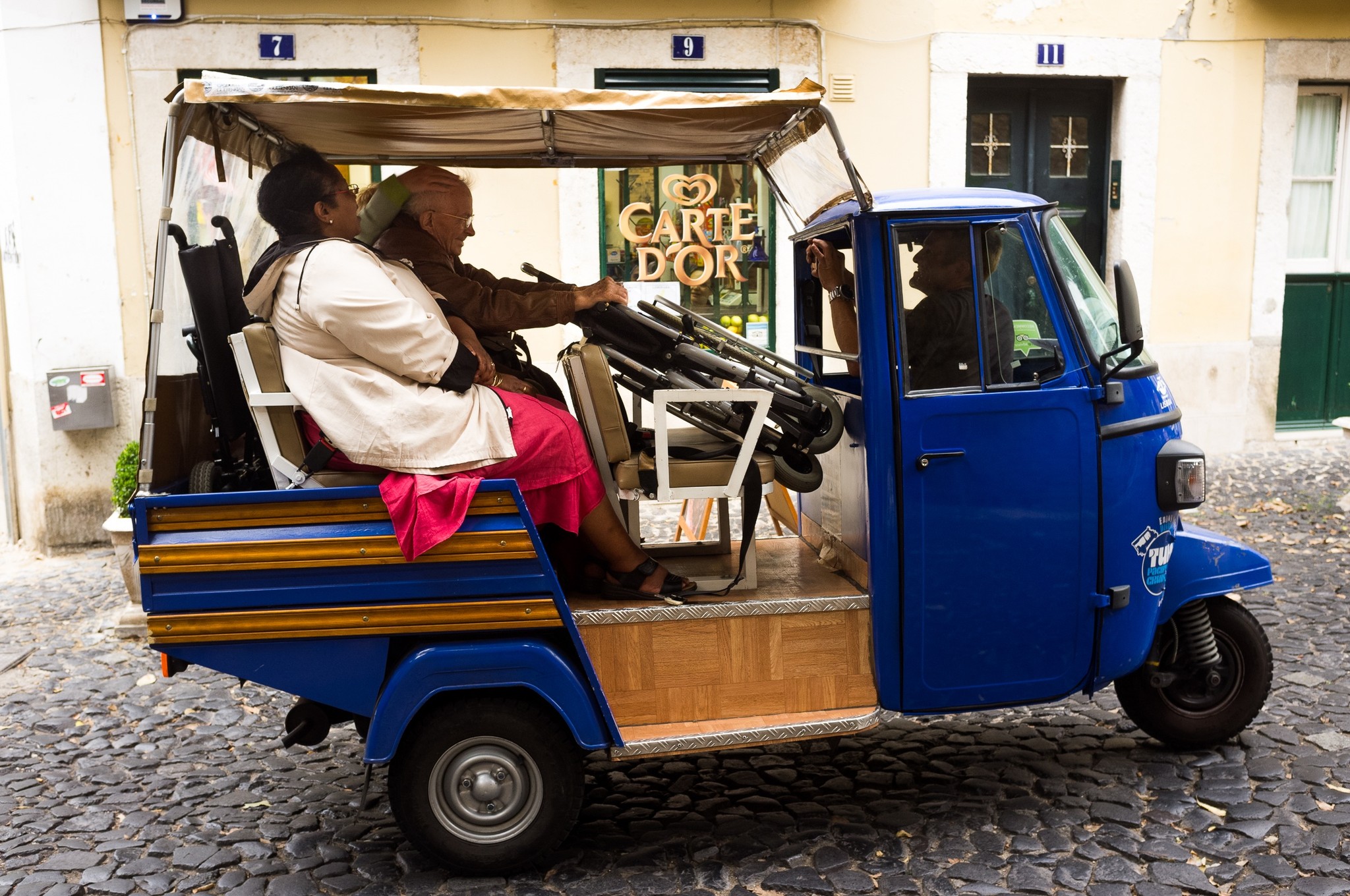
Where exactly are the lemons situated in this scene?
[690,314,768,349]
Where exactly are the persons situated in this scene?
[240,144,699,600]
[804,224,1016,392]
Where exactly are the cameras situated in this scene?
[815,257,819,270]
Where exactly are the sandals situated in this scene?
[594,555,697,600]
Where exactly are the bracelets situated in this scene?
[490,372,497,386]
[495,374,501,387]
[499,376,503,385]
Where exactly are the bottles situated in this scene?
[672,197,683,234]
[729,196,742,226]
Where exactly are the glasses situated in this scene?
[430,210,475,228]
[314,184,359,204]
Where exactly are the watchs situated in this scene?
[828,283,855,304]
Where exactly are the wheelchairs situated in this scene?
[520,261,845,494]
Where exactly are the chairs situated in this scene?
[563,334,776,593]
[228,323,388,488]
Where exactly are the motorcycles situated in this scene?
[127,74,1278,879]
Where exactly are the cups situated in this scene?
[723,237,743,262]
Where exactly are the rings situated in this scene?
[490,362,495,367]
[522,386,527,392]
